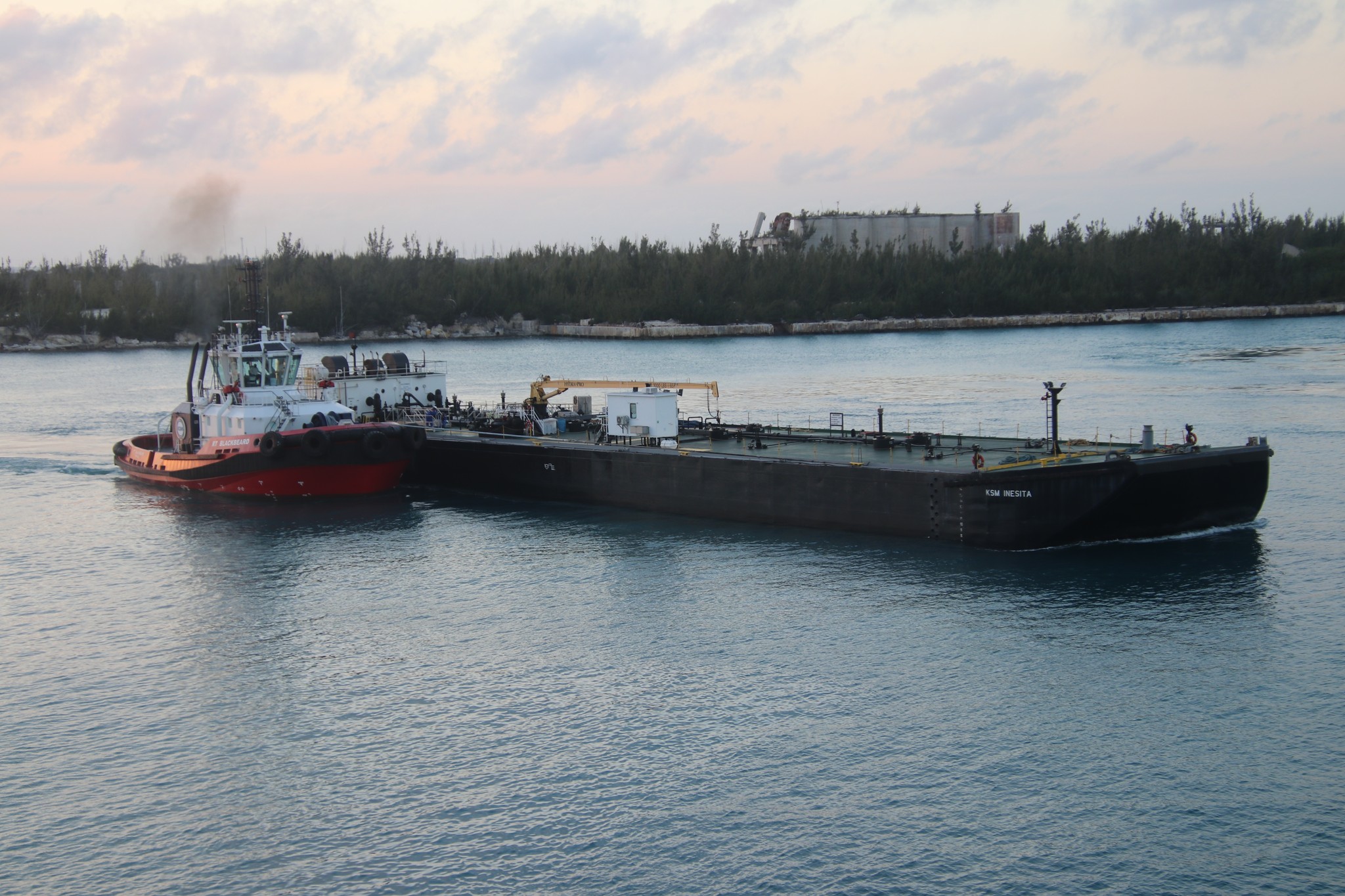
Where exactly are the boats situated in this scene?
[112,312,429,511]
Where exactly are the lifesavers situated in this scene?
[972,454,985,467]
[1186,433,1197,445]
[301,424,426,456]
[260,431,283,456]
[524,421,532,430]
[238,393,246,403]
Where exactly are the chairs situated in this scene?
[249,366,258,384]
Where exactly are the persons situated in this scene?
[249,364,262,381]
[425,406,443,426]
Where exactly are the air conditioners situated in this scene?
[616,416,627,424]
